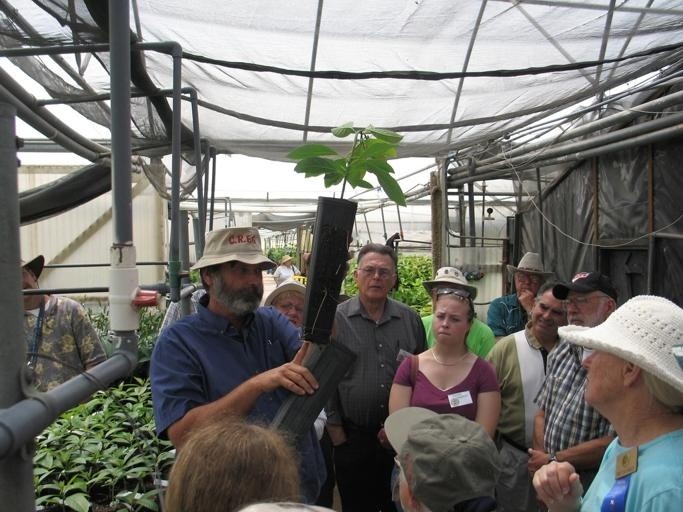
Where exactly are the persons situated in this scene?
[528,269,618,497]
[150,227,329,504]
[422,253,555,356]
[273,255,301,286]
[168,418,297,512]
[532,295,683,511]
[329,244,429,511]
[388,287,499,440]
[486,278,573,511]
[265,278,335,511]
[21,255,108,392]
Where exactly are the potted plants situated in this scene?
[30,386,160,512]
[284,115,408,341]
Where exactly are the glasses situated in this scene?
[563,294,609,311]
[514,272,539,285]
[436,288,474,317]
[358,266,394,280]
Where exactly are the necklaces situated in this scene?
[430,349,470,364]
[26,295,45,370]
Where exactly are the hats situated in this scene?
[264,278,306,306]
[552,271,617,302]
[20,255,45,281]
[190,227,276,271]
[282,255,294,264]
[422,266,478,300]
[507,252,550,278]
[558,295,683,392]
